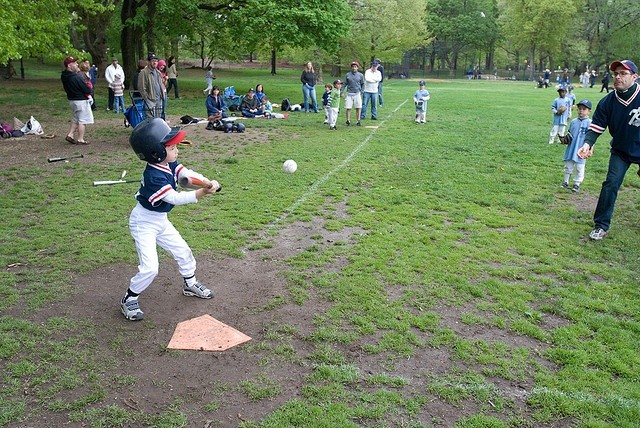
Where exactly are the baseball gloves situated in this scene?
[557,130,573,144]
[557,106,567,116]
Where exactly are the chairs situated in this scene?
[129,90,181,133]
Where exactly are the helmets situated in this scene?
[129,118,186,163]
[206,120,246,132]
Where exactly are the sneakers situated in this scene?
[590,227,610,240]
[182,281,214,299]
[330,125,336,129]
[573,184,579,191]
[346,121,350,125]
[120,298,144,322]
[77,141,90,144]
[357,122,361,125]
[65,136,76,143]
[561,182,568,187]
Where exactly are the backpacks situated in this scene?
[281,99,292,111]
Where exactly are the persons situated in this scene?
[108,73,126,112]
[327,80,344,130]
[590,68,598,89]
[492,64,499,80]
[583,68,591,88]
[547,85,570,144]
[201,65,217,96]
[165,56,180,99]
[237,88,262,118]
[599,68,611,94]
[578,72,583,84]
[559,99,592,194]
[257,97,273,117]
[339,62,366,127]
[375,58,385,108]
[298,61,319,113]
[558,68,573,90]
[137,53,165,119]
[564,86,576,118]
[118,116,220,322]
[538,75,548,88]
[122,60,147,128]
[576,58,640,242]
[255,83,265,106]
[78,60,95,105]
[359,60,382,121]
[158,59,169,120]
[543,69,552,86]
[206,85,228,122]
[413,79,430,125]
[61,54,97,147]
[322,82,334,124]
[466,65,473,80]
[474,64,482,79]
[104,57,125,111]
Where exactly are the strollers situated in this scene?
[123,90,154,129]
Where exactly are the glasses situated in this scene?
[612,72,634,77]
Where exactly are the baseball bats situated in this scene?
[47,154,84,162]
[179,176,222,192]
[93,180,142,185]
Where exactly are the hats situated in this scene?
[63,56,78,65]
[334,80,344,84]
[557,85,567,90]
[419,80,426,85]
[148,53,159,59]
[611,60,639,74]
[112,56,117,60]
[351,62,358,66]
[249,87,255,91]
[577,100,592,109]
